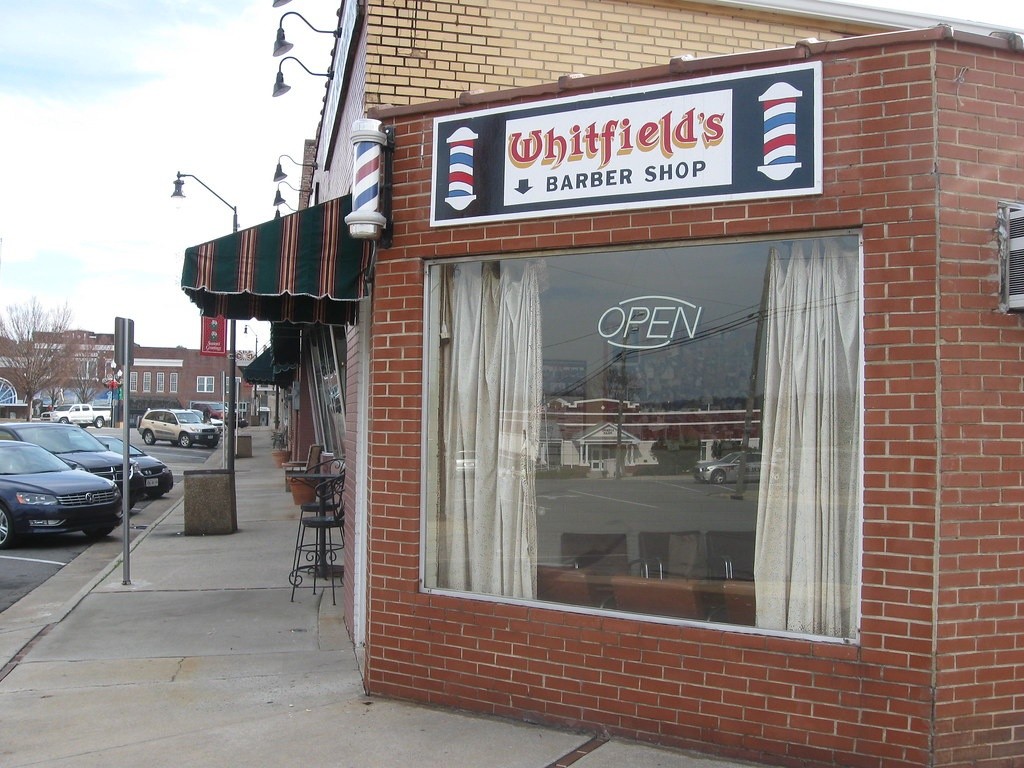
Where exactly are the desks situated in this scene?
[288,473,345,578]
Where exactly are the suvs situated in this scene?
[192,401,238,428]
[0,422,143,512]
[138,407,220,449]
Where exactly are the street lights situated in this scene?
[111,358,117,428]
[170,170,238,536]
[244,325,259,426]
[117,369,123,422]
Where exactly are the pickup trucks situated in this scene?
[41,403,113,429]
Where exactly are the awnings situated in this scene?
[244,344,297,390]
[268,320,301,383]
[181,194,371,327]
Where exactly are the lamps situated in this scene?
[272,12,339,57]
[272,56,333,97]
[273,1,294,8]
[274,202,298,222]
[274,180,313,206]
[346,118,397,249]
[273,154,319,183]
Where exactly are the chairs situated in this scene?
[285,444,323,493]
[288,473,346,606]
[292,457,347,572]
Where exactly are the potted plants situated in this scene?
[271,429,291,468]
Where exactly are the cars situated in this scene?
[455,449,476,477]
[694,450,763,484]
[184,409,224,434]
[1,440,124,549]
[91,436,174,498]
